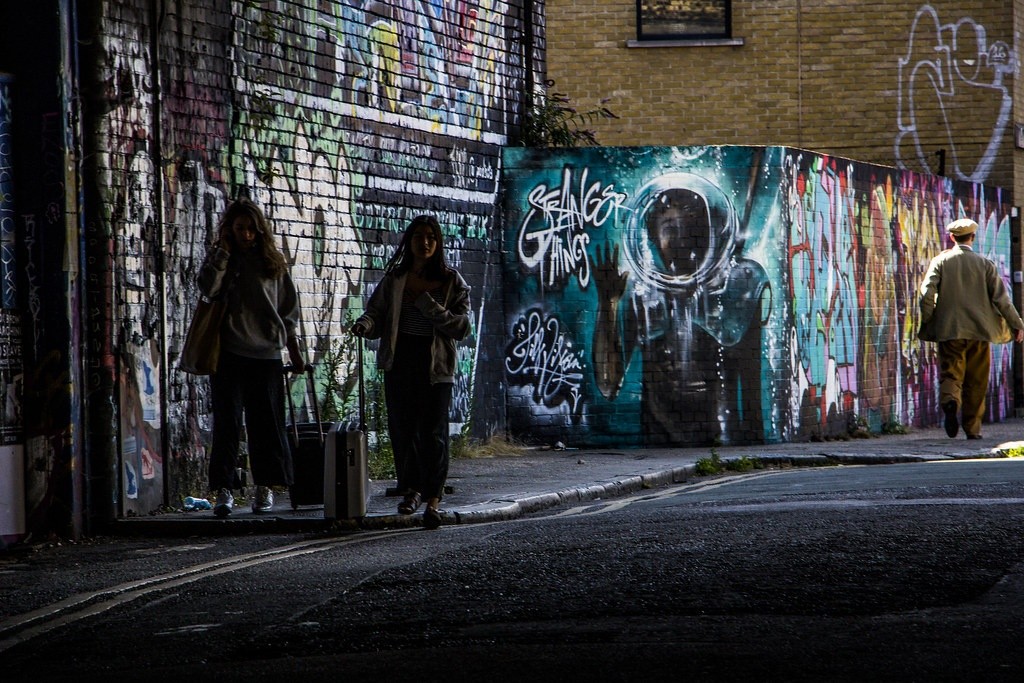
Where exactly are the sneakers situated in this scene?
[252,486,274,513]
[214,488,234,517]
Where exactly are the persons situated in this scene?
[351,214,472,526]
[916,219,1023,440]
[197,198,304,515]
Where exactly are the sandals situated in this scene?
[398,492,422,514]
[424,508,441,528]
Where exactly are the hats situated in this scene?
[947,219,978,236]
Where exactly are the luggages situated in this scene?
[283,363,335,510]
[324,332,370,530]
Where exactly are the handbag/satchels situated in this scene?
[918,261,942,342]
[179,276,232,375]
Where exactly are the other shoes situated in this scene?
[944,400,959,438]
[967,435,982,439]
